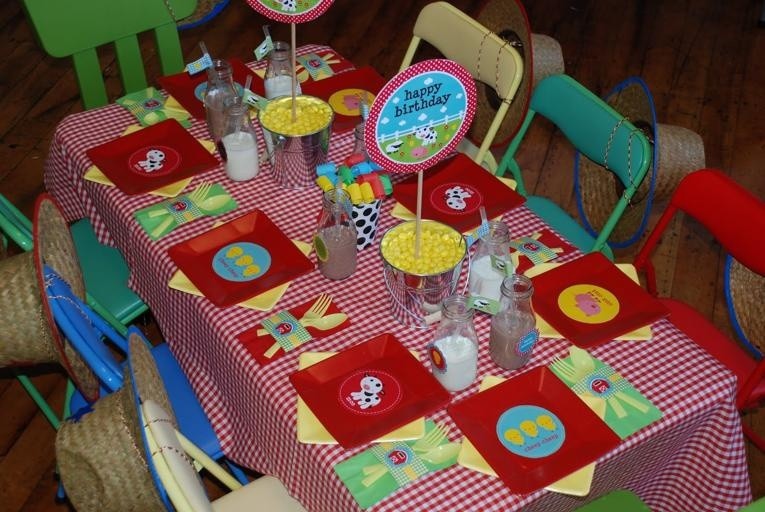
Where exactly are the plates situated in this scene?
[153,58,264,120]
[296,351,424,444]
[301,65,389,135]
[534,261,653,341]
[289,332,452,451]
[392,152,527,233]
[447,364,623,497]
[166,210,316,308]
[169,220,313,312]
[457,375,606,496]
[392,176,517,236]
[528,249,673,349]
[162,65,265,122]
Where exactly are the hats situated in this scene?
[55,325,179,511]
[726,250,764,358]
[576,81,705,248]
[464,1,566,148]
[0,193,101,403]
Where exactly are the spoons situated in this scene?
[148,194,230,220]
[365,443,461,474]
[257,314,347,335]
[567,344,627,418]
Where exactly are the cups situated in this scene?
[351,200,382,254]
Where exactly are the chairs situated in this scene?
[22,1,214,122]
[129,390,312,512]
[38,266,244,502]
[628,166,764,461]
[385,1,525,182]
[0,182,158,328]
[490,72,657,265]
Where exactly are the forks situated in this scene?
[150,179,213,238]
[551,355,648,413]
[361,424,450,486]
[265,292,332,357]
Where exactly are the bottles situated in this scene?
[490,272,538,372]
[205,61,240,139]
[317,189,359,280]
[217,97,261,182]
[468,221,513,316]
[263,40,299,101]
[353,122,367,154]
[432,296,477,392]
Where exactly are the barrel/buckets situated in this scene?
[379,218,471,329]
[260,94,335,190]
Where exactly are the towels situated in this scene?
[261,43,349,93]
[502,223,580,273]
[542,342,668,448]
[231,292,357,373]
[109,81,194,137]
[129,182,246,245]
[327,413,465,512]
